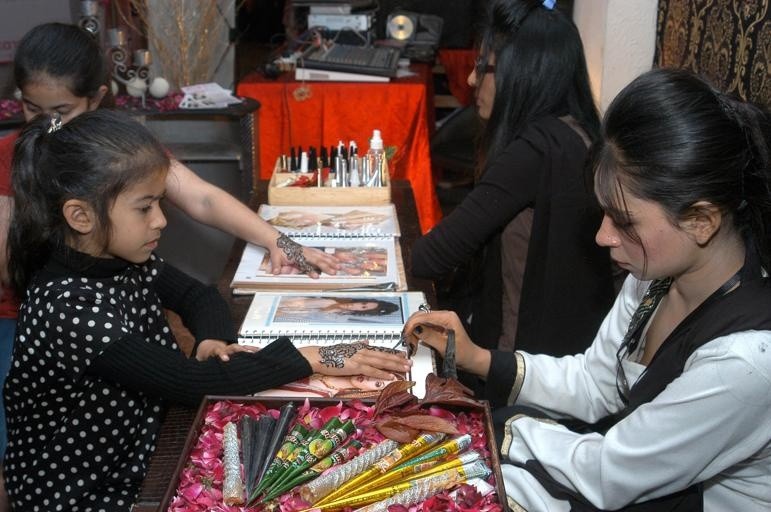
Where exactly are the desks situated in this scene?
[127,179,442,510]
[233,44,432,184]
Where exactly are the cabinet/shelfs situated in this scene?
[0,96,260,205]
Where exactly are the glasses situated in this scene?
[475,56,496,80]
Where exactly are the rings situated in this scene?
[419,303,432,314]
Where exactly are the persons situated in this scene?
[399,68,771,512]
[275,294,399,317]
[0,23,342,466]
[258,370,406,399]
[402,0,616,357]
[3,105,413,511]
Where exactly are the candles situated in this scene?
[106,27,151,67]
[79,0,98,16]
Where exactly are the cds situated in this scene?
[387,16,413,40]
